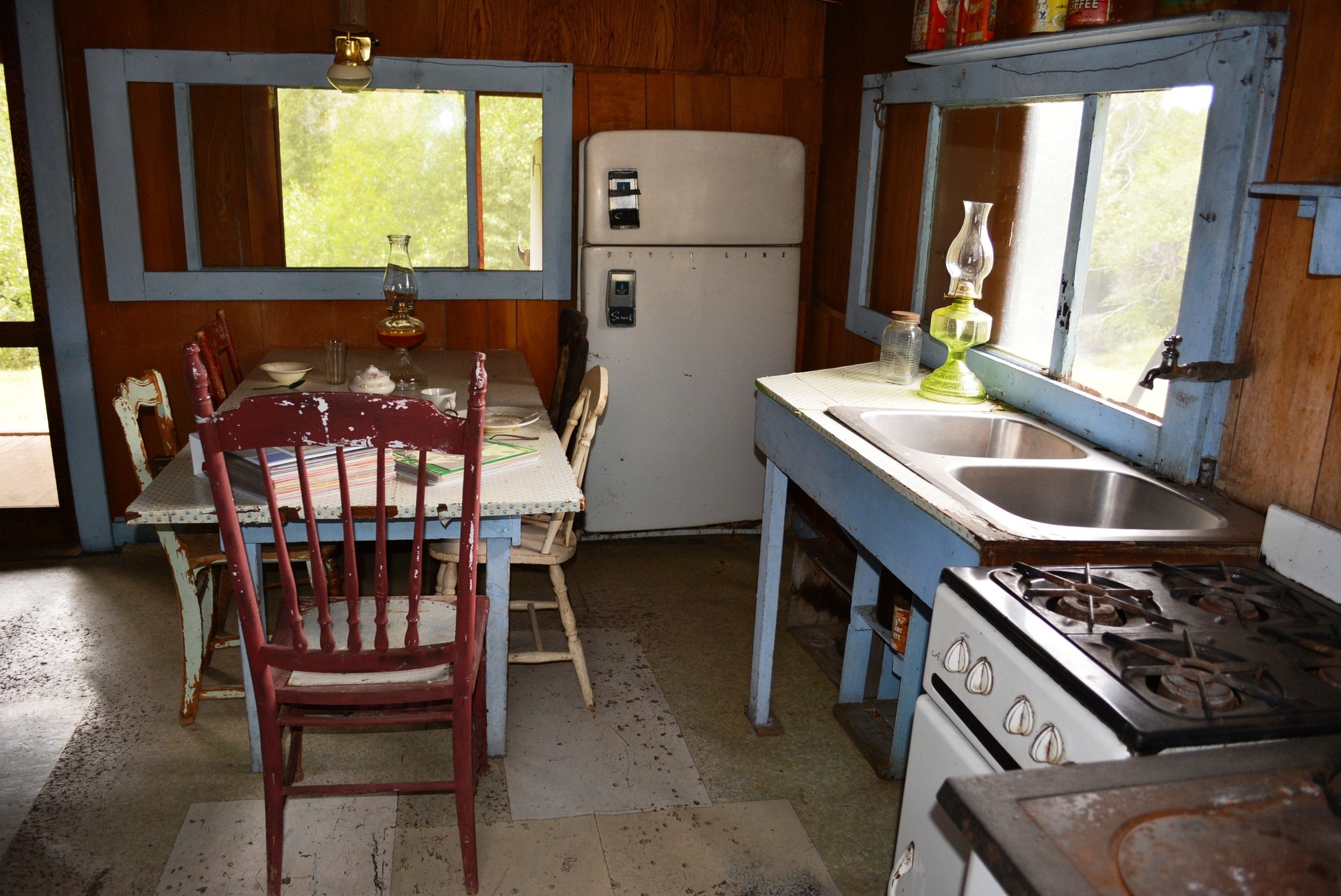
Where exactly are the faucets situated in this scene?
[1138,334,1251,390]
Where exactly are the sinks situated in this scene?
[903,462,1267,542]
[827,406,1133,462]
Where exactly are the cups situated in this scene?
[891,603,911,653]
[421,387,456,413]
[324,339,347,384]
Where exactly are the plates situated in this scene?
[451,407,540,433]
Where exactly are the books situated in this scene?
[391,438,539,486]
[223,446,398,504]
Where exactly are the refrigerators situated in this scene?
[572,129,807,540]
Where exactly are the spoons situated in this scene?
[253,380,306,390]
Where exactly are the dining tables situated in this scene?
[125,345,587,758]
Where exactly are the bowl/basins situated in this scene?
[260,361,315,383]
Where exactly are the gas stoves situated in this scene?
[924,502,1341,778]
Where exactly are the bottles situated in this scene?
[879,310,923,385]
[910,0,1230,51]
[349,365,395,396]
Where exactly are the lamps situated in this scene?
[916,197,997,404]
[327,25,379,96]
[378,234,428,390]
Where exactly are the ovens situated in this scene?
[886,693,998,896]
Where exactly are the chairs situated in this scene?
[548,305,590,436]
[110,309,608,896]
